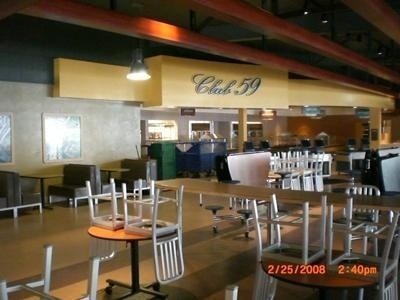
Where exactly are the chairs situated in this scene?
[87,149,399,300]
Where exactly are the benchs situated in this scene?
[0,159,158,221]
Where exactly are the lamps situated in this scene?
[126,37,152,82]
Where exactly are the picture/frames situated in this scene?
[0,112,82,164]
